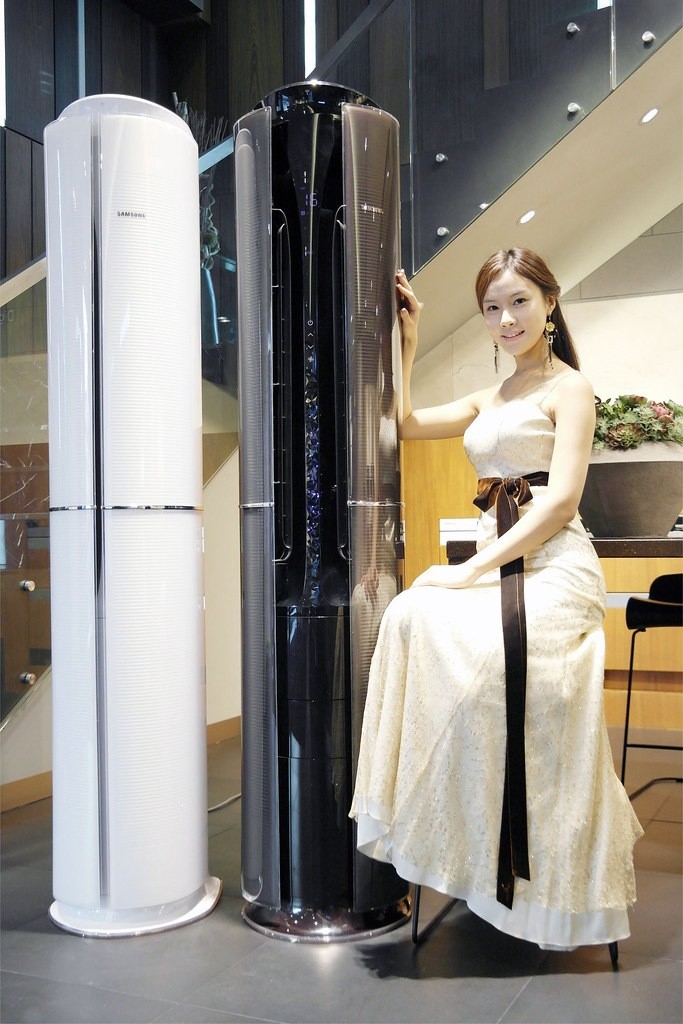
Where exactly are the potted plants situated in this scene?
[574,394,683,538]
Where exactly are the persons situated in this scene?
[347,246,644,952]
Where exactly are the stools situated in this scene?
[621,572,683,803]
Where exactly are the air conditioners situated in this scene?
[44,94,224,937]
[237,80,415,943]
